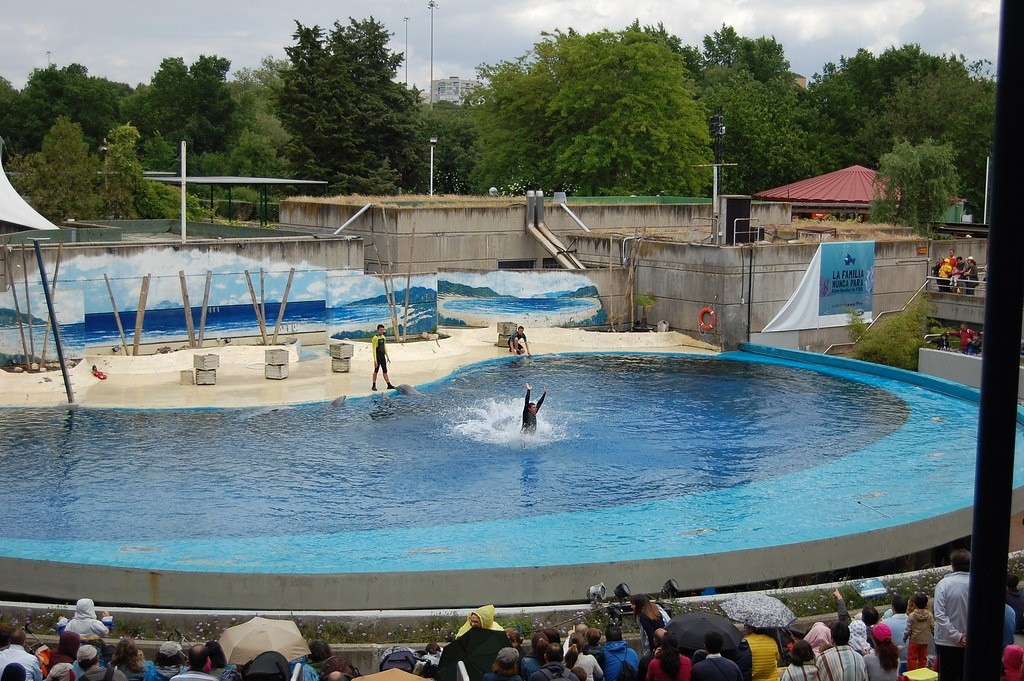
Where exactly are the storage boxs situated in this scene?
[496,322,518,335]
[329,343,354,360]
[498,334,511,347]
[193,353,220,372]
[265,349,289,366]
[196,368,217,386]
[265,363,289,380]
[332,357,351,373]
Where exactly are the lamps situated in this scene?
[237,242,245,249]
[613,582,631,600]
[112,346,119,353]
[590,582,607,601]
[109,247,115,255]
[663,578,679,596]
[224,337,232,343]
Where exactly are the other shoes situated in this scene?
[371,386,395,391]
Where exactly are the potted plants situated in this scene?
[631,291,656,332]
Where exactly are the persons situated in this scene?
[520,382,547,434]
[932,248,979,294]
[925,323,982,354]
[0,550,1024,681]
[371,324,395,391]
[508,326,531,357]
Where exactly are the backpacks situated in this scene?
[539,667,572,681]
[610,646,638,681]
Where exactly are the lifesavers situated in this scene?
[699,307,716,331]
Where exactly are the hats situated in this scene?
[159,641,182,657]
[242,651,291,681]
[77,645,98,662]
[869,623,892,642]
[496,647,519,669]
[967,256,974,260]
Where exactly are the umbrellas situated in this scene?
[436,627,513,681]
[662,611,745,653]
[717,591,797,631]
[219,616,313,666]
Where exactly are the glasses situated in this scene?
[944,261,948,263]
[968,261,971,264]
